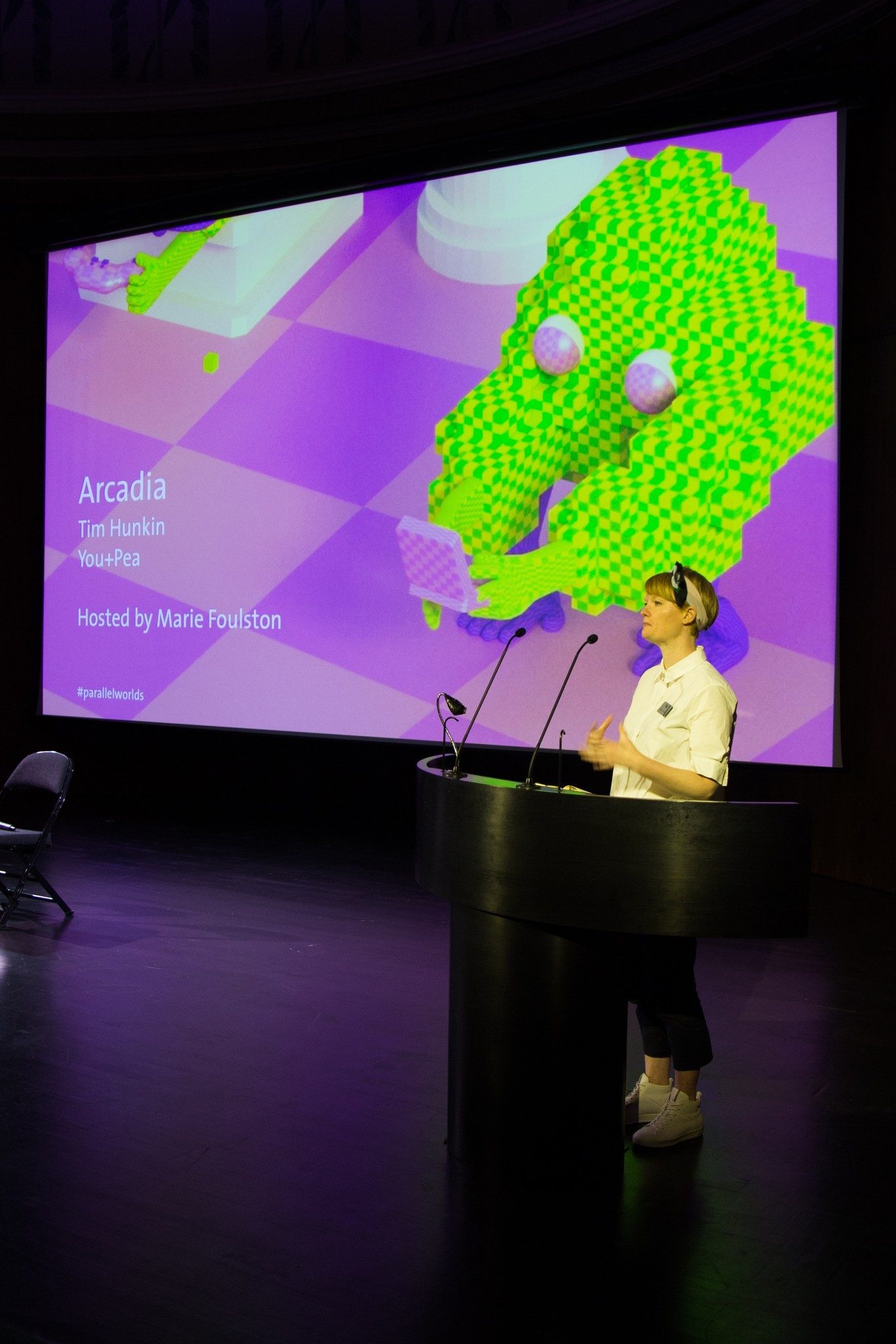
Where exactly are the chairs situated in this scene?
[0,750,74,929]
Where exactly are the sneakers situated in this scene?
[632,1087,705,1148]
[624,1073,673,1125]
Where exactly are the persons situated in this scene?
[579,562,738,1149]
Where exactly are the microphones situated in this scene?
[447,627,526,777]
[517,634,598,791]
[436,693,466,772]
